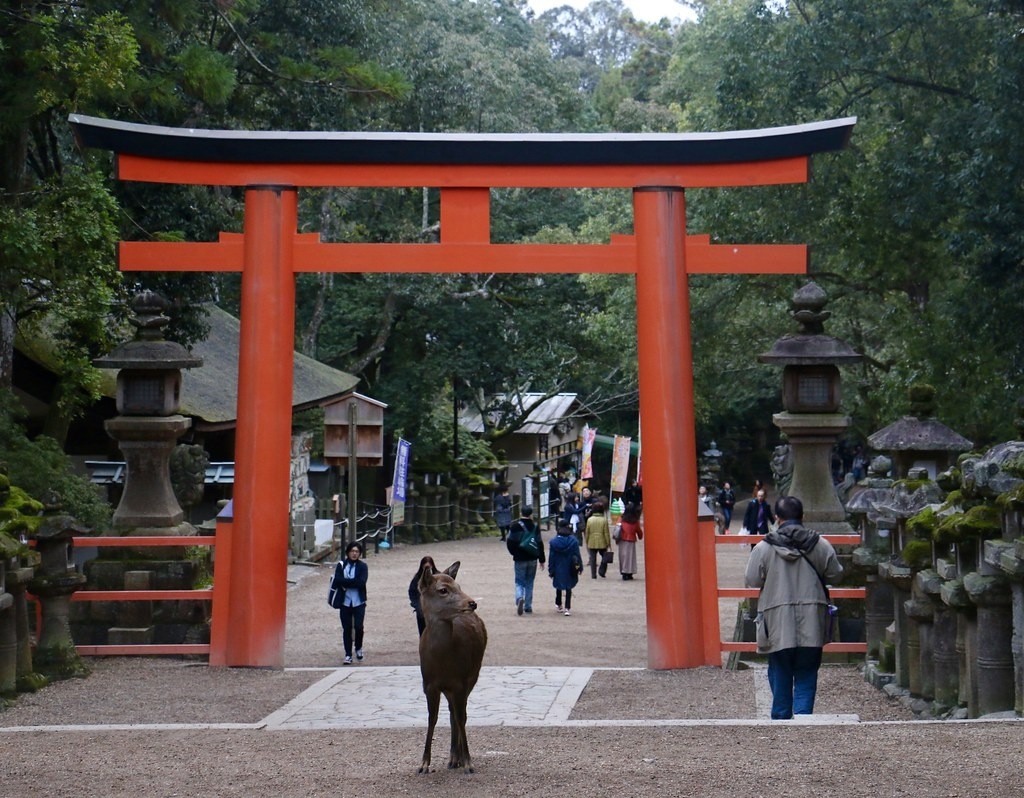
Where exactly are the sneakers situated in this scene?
[343,655,352,664]
[564,608,572,616]
[355,649,364,661]
[517,598,524,615]
[556,605,563,613]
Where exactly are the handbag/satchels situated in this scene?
[826,605,838,643]
[327,576,346,608]
[604,552,614,563]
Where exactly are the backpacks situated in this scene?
[518,521,543,557]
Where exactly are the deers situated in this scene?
[415,560,487,775]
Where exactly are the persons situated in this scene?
[744,496,845,720]
[494,485,514,542]
[698,485,715,513]
[585,503,611,578]
[611,478,643,539]
[743,488,777,552]
[548,520,584,616]
[409,557,442,637]
[614,503,643,581]
[334,542,368,665]
[718,481,736,535]
[547,470,601,565]
[751,480,769,501]
[507,506,545,617]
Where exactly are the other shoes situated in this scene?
[500,537,507,543]
[623,574,628,580]
[628,573,633,579]
[724,529,729,535]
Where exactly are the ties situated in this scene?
[758,503,763,528]
